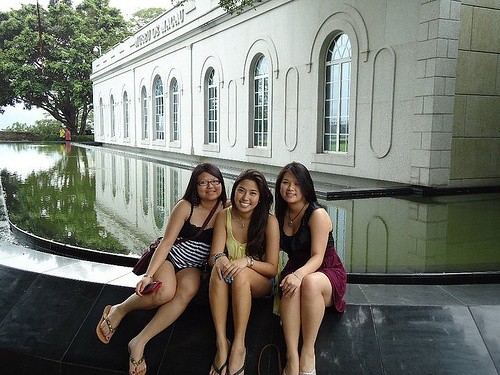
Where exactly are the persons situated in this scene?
[97,164,231,375]
[275,164,347,375]
[209,169,280,375]
[59,126,71,142]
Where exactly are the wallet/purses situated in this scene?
[141,281,163,294]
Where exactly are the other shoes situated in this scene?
[282,368,286,375]
[299,368,316,375]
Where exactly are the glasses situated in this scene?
[196,179,221,186]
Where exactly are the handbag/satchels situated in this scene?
[132,237,179,276]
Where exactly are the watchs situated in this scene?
[214,253,226,262]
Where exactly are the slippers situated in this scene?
[209,336,231,375]
[96,305,117,344]
[128,345,147,375]
[228,343,248,375]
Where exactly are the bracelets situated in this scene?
[143,275,152,279]
[293,273,302,281]
[248,256,254,268]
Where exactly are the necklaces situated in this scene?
[287,201,307,226]
[239,217,247,227]
